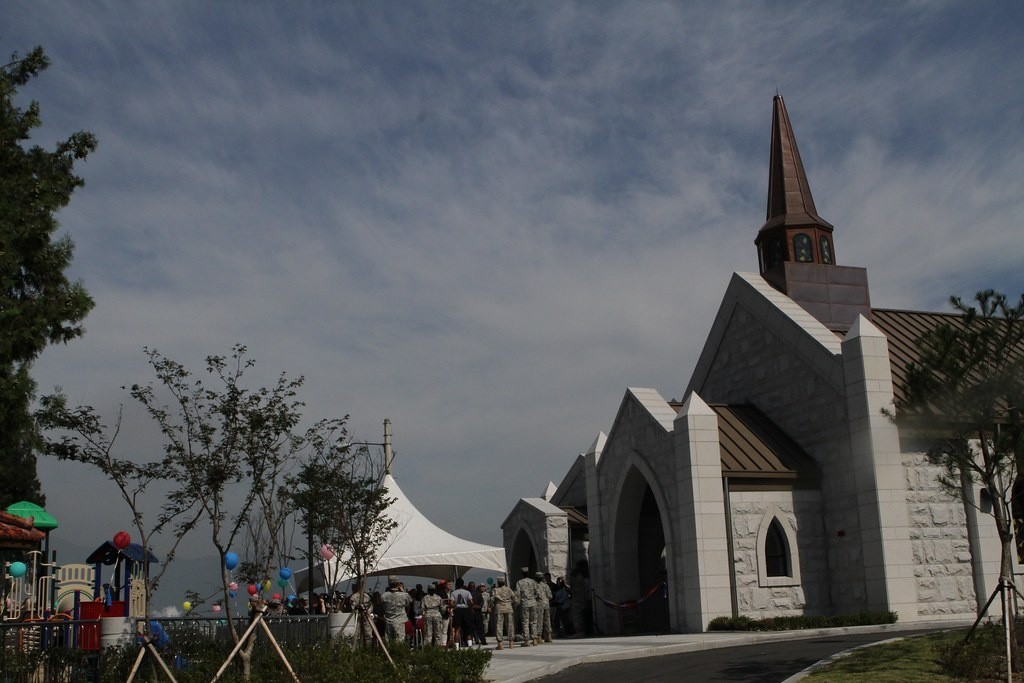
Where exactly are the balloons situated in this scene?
[320,544,335,560]
[182,552,296,612]
[9,562,26,578]
[114,531,132,549]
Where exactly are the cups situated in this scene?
[453,643,459,650]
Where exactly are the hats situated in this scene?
[428,584,435,589]
[536,572,543,577]
[497,577,505,582]
[521,567,529,572]
[480,583,485,587]
[387,575,400,584]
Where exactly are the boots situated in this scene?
[509,641,513,648]
[537,636,543,643]
[521,640,530,647]
[545,634,552,642]
[495,642,504,650]
[533,638,537,646]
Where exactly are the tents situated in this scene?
[295,471,507,592]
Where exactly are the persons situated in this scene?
[382,575,412,648]
[248,567,576,651]
[515,567,541,647]
[490,576,518,650]
